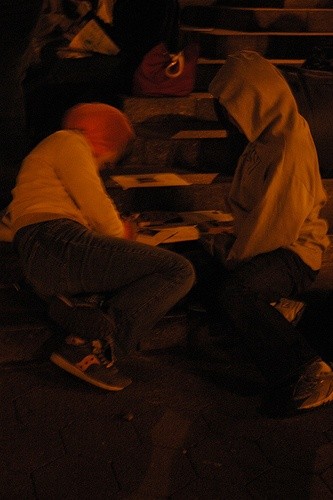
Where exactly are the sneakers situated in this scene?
[49,336,132,391]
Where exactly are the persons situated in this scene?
[204,49,333,416]
[8,104,197,391]
[17,1,196,175]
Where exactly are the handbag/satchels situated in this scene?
[133,39,197,97]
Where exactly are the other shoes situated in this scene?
[288,366,333,412]
